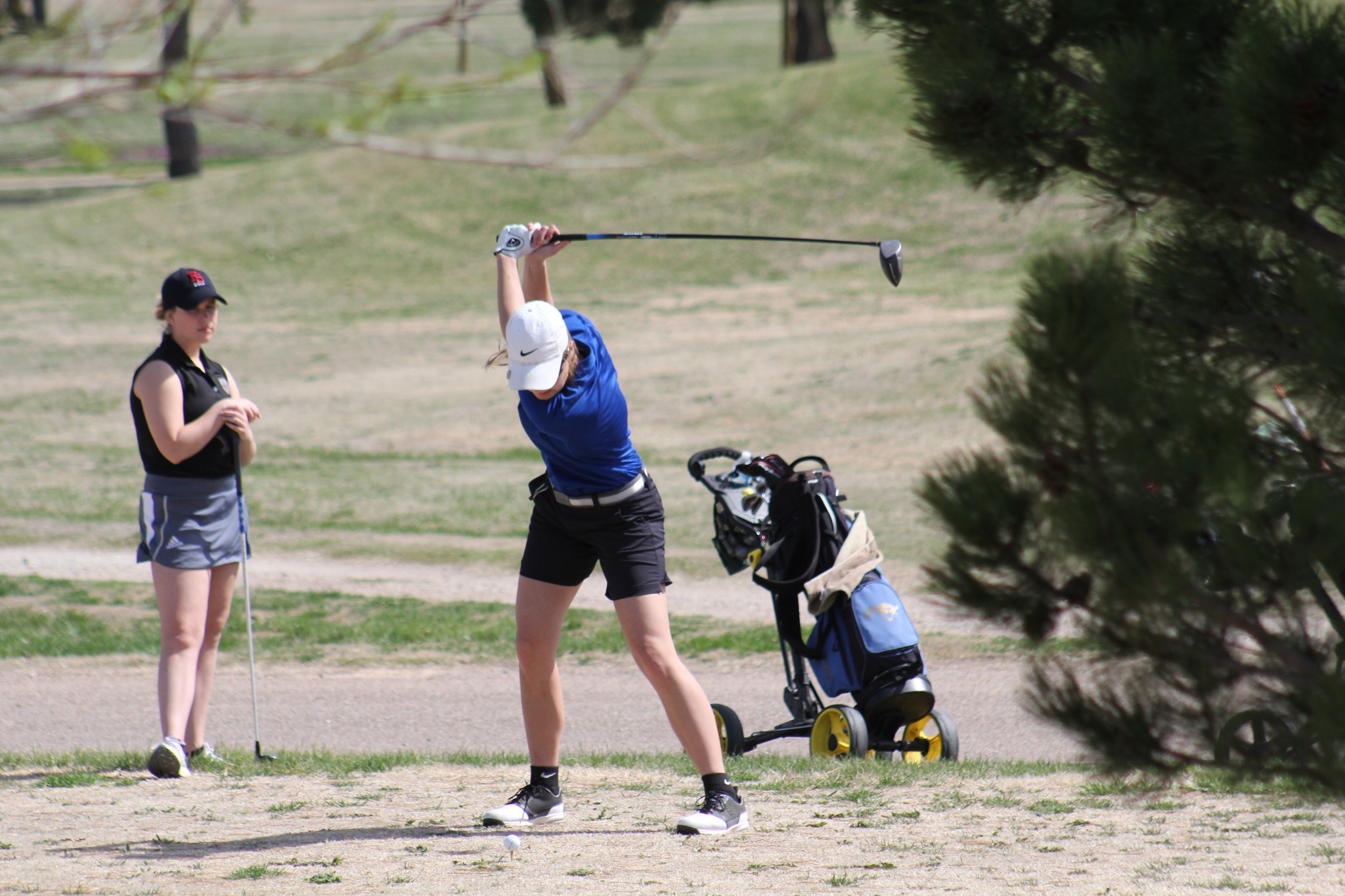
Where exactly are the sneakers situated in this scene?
[147,736,191,778]
[483,781,564,827]
[185,743,235,767]
[676,786,749,835]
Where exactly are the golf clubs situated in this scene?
[231,425,279,763]
[552,232,905,288]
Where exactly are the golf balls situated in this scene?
[503,834,520,851]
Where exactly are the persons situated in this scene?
[129,267,261,781]
[483,223,747,834]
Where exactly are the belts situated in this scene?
[547,458,648,508]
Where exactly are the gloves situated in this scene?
[493,221,545,260]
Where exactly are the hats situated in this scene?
[505,300,569,391]
[162,268,228,311]
